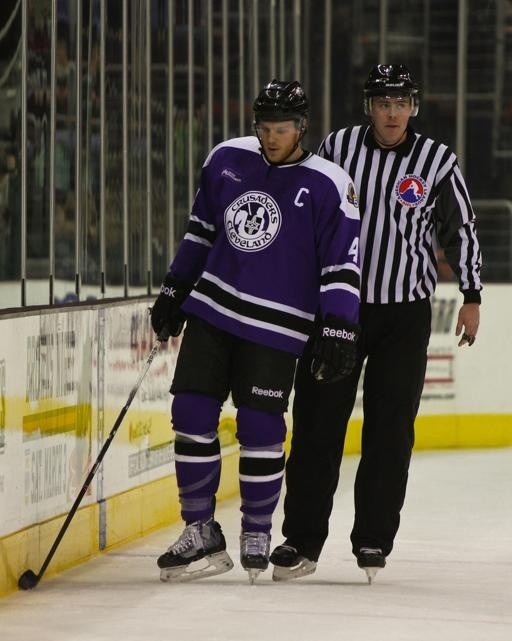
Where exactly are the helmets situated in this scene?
[254,80,311,122]
[365,64,423,117]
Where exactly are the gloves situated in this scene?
[148,271,195,343]
[311,314,362,382]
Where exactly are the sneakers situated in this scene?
[158,519,226,569]
[355,547,386,566]
[270,543,320,566]
[240,532,272,568]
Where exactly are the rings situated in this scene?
[461,332,476,348]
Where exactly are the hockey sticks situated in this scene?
[17,335,163,591]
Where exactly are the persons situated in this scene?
[269,62,484,570]
[1,1,265,287]
[150,76,360,570]
